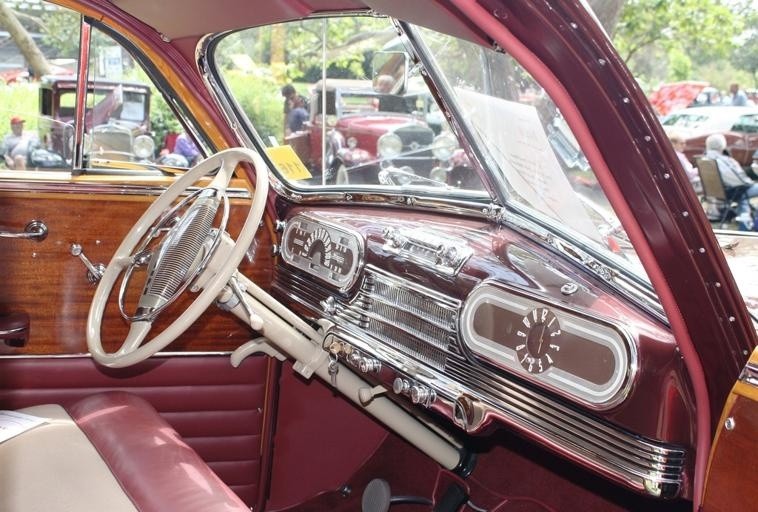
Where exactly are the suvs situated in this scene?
[285,78,481,191]
[24,73,189,169]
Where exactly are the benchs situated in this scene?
[0,392,255,511]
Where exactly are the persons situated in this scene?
[0,116,41,171]
[284,96,309,137]
[374,74,396,93]
[660,81,758,232]
[281,85,309,120]
[154,127,202,169]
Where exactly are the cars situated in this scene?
[644,80,758,177]
[0,65,73,86]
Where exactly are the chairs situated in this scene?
[695,158,753,231]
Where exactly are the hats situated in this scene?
[11,117,25,126]
